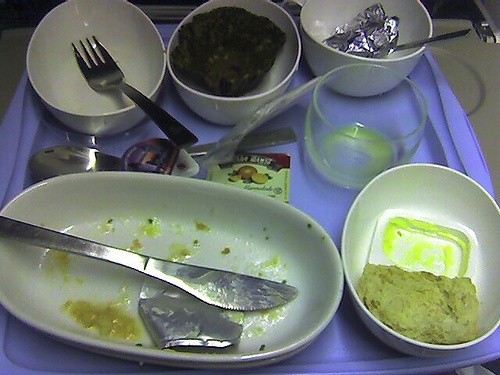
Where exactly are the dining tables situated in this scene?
[0,19,500,374]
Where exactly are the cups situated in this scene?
[305,62,430,190]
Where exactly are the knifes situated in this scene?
[0,215,301,319]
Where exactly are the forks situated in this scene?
[68,35,198,148]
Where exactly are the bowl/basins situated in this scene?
[166,0,304,127]
[341,162,500,357]
[26,0,169,136]
[299,0,432,96]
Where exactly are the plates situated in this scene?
[0,166,346,369]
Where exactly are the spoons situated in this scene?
[124,140,209,186]
[28,118,298,183]
[348,26,472,61]
